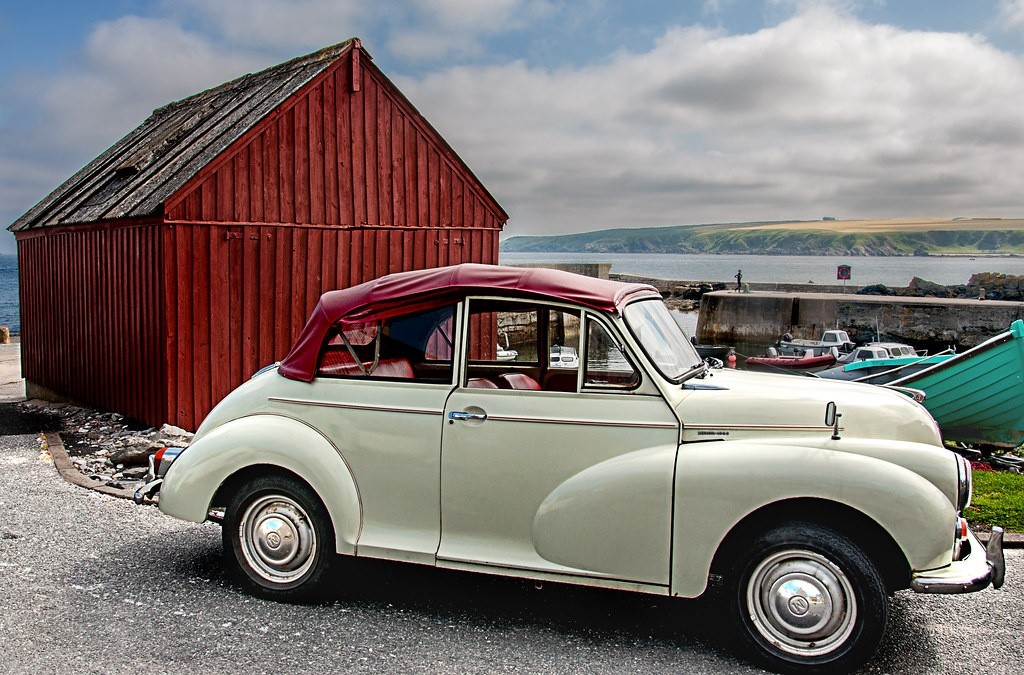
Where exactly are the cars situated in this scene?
[132,260,1005,675]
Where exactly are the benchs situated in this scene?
[320,357,415,379]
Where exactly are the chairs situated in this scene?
[500,372,541,390]
[466,378,499,389]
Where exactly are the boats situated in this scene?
[689,336,736,362]
[742,347,838,373]
[837,345,890,364]
[780,329,856,356]
[866,315,929,358]
[548,346,579,369]
[802,319,1024,448]
[497,342,518,361]
